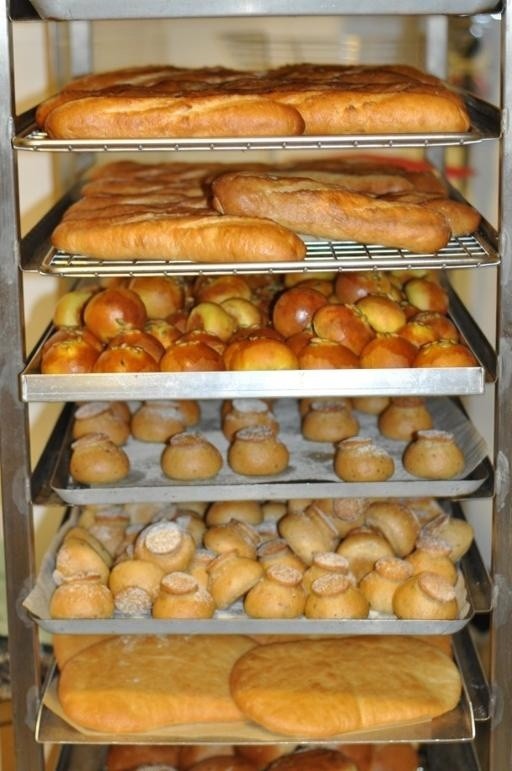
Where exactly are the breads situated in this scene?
[47,497,481,620]
[66,393,468,484]
[103,742,426,771]
[49,631,463,737]
[36,54,475,143]
[33,271,475,376]
[46,160,481,265]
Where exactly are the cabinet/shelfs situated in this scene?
[0,0,512,770]
